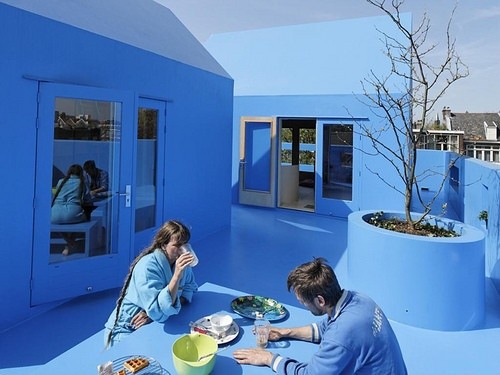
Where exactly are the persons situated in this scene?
[51,163,87,255]
[83,160,109,195]
[103,220,198,347]
[234,256,408,375]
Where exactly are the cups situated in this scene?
[172,332,218,375]
[206,312,233,332]
[254,319,270,348]
[178,243,199,267]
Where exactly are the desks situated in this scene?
[51,188,109,229]
[70,281,325,375]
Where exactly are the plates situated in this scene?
[230,296,287,321]
[190,315,239,345]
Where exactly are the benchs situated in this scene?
[50,220,98,257]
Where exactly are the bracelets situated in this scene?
[271,353,280,372]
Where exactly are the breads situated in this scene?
[124,357,149,372]
[117,370,126,375]
